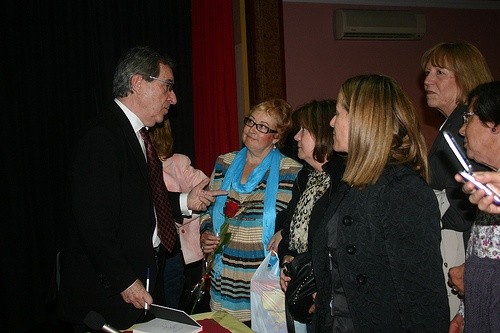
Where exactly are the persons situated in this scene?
[145,118,213,317]
[454,168,499,218]
[419,40,496,313]
[264,98,349,333]
[448,77,500,333]
[199,97,304,329]
[49,45,190,333]
[302,72,452,333]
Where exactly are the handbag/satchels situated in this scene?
[286,252,316,324]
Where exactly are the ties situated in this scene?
[139,127,178,253]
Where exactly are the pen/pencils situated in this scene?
[144,267,150,316]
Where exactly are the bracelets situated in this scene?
[456,299,467,319]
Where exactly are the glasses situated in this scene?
[462,111,475,122]
[149,75,175,92]
[244,117,278,134]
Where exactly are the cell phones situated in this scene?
[442,130,500,205]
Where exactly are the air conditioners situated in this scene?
[332,9,427,42]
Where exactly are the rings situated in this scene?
[452,286,458,293]
